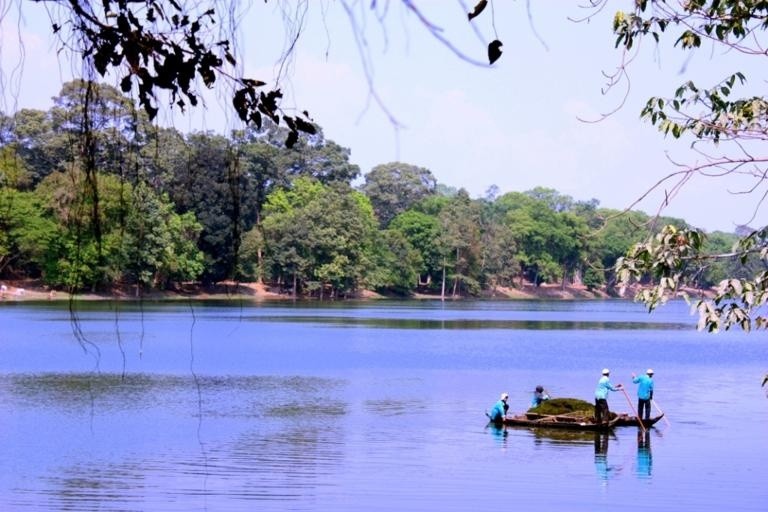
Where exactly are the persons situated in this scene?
[633,369,654,420]
[491,423,508,452]
[635,426,653,480]
[531,385,549,409]
[534,435,542,446]
[596,428,622,486]
[594,368,623,422]
[491,393,509,422]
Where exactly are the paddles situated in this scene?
[622,387,646,431]
[635,375,671,428]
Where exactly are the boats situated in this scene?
[504,411,621,429]
[620,413,665,426]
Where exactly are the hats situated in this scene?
[647,370,653,374]
[501,393,509,400]
[603,369,609,375]
